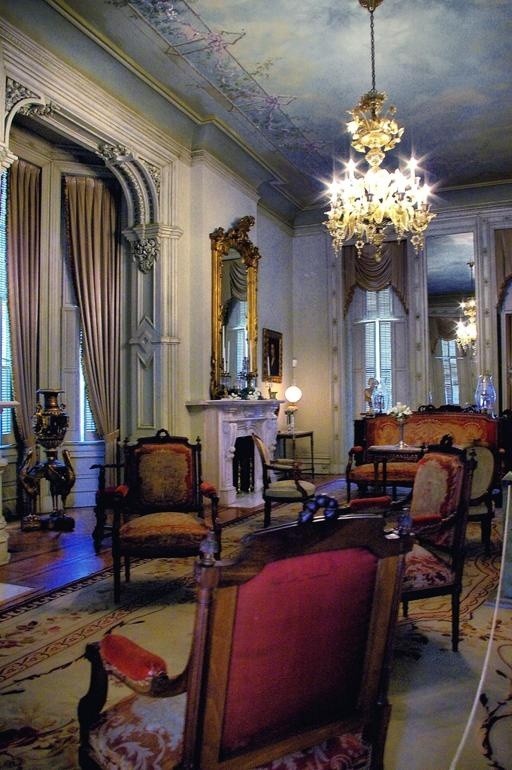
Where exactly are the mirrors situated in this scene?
[210,216,261,400]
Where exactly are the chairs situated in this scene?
[77,491,417,770]
[113,427,222,604]
[330,442,477,650]
[90,436,129,554]
[252,432,317,529]
[450,447,495,554]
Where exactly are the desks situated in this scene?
[276,430,315,481]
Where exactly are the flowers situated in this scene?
[285,405,298,424]
[388,402,413,423]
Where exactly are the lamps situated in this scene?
[454,262,477,355]
[284,385,302,404]
[320,0,439,262]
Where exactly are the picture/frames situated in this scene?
[262,327,285,383]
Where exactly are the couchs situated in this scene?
[345,404,504,509]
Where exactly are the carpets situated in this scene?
[0,480,512,770]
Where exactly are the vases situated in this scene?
[394,421,409,447]
[32,388,69,448]
[286,415,295,431]
